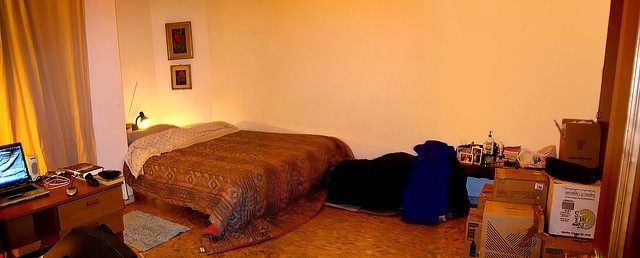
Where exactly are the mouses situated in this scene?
[66,186,78,196]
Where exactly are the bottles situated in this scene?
[497,143,504,156]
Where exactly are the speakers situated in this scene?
[28,156,40,182]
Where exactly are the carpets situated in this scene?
[123,210,191,253]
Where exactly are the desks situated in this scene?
[0,164,126,258]
[457,161,546,179]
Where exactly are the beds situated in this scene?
[122,122,355,255]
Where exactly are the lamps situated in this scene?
[131,111,147,131]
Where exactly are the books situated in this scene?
[58,162,104,179]
[503,145,522,160]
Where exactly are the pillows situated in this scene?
[127,124,178,143]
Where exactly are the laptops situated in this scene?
[0,142,50,207]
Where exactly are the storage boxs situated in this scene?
[493,167,551,211]
[477,183,494,218]
[553,118,601,169]
[546,178,600,239]
[479,200,544,258]
[464,207,483,258]
[542,235,594,258]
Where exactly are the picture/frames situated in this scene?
[459,153,473,164]
[472,147,482,165]
[169,64,192,90]
[165,21,194,60]
